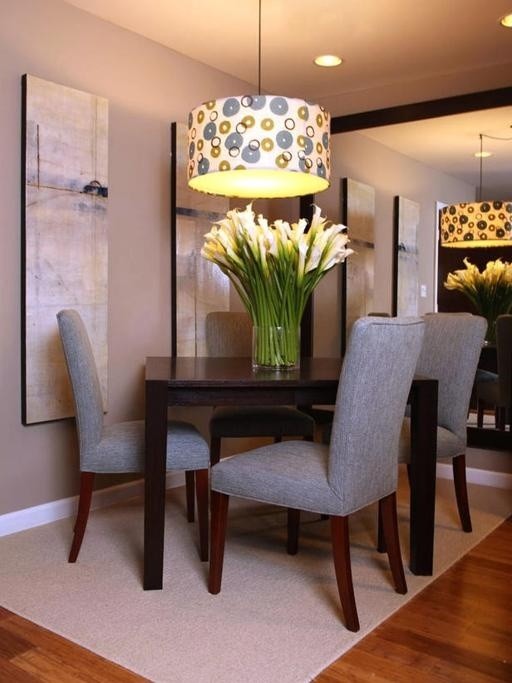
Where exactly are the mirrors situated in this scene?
[299,87,511,460]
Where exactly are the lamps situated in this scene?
[188,2,330,201]
[438,132,511,249]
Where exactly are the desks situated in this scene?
[138,353,445,592]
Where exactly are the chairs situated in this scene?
[374,310,486,555]
[476,314,512,431]
[201,310,318,470]
[57,307,211,567]
[205,315,427,631]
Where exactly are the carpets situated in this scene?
[1,483,507,682]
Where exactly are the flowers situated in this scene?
[444,255,512,321]
[200,203,357,368]
